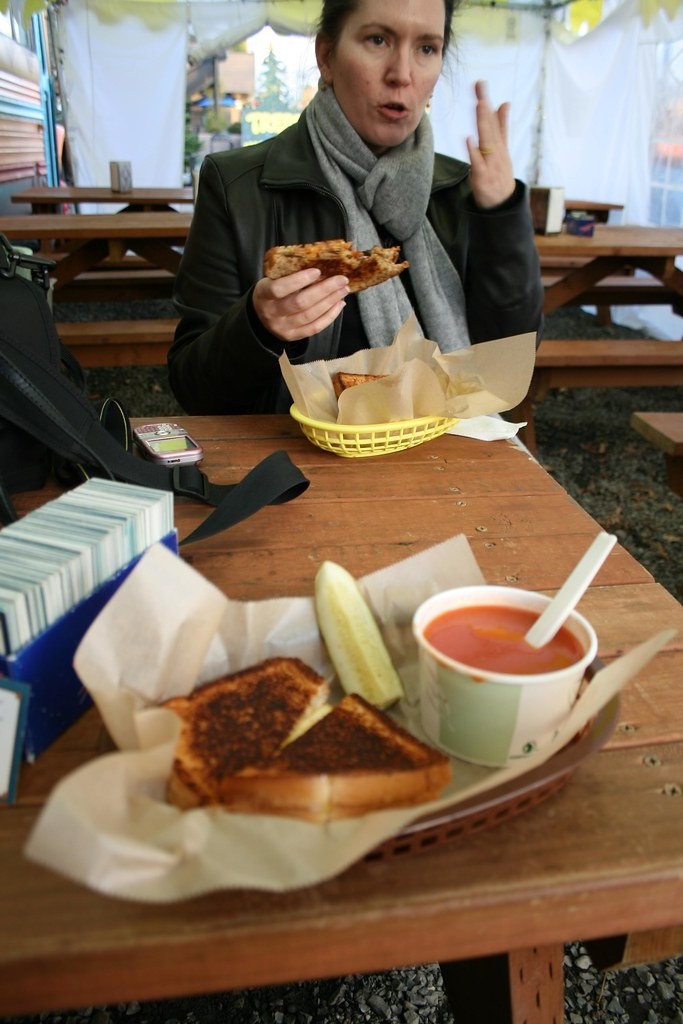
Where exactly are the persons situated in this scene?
[164,0,545,1024]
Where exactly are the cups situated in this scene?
[412,585,599,768]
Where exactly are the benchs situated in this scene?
[539,275,671,325]
[631,412,683,456]
[74,270,195,288]
[532,340,683,393]
[35,252,167,270]
[54,319,176,365]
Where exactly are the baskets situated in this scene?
[290,401,459,456]
[348,658,622,871]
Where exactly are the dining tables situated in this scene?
[0,416,683,1024]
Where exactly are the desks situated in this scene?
[564,201,625,224]
[533,228,683,314]
[0,216,189,251]
[9,187,193,214]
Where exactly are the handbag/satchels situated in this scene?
[0,234,86,492]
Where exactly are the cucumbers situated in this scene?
[312,559,406,713]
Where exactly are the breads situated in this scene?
[263,237,411,293]
[334,371,388,397]
[150,656,454,824]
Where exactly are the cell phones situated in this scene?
[133,422,204,468]
